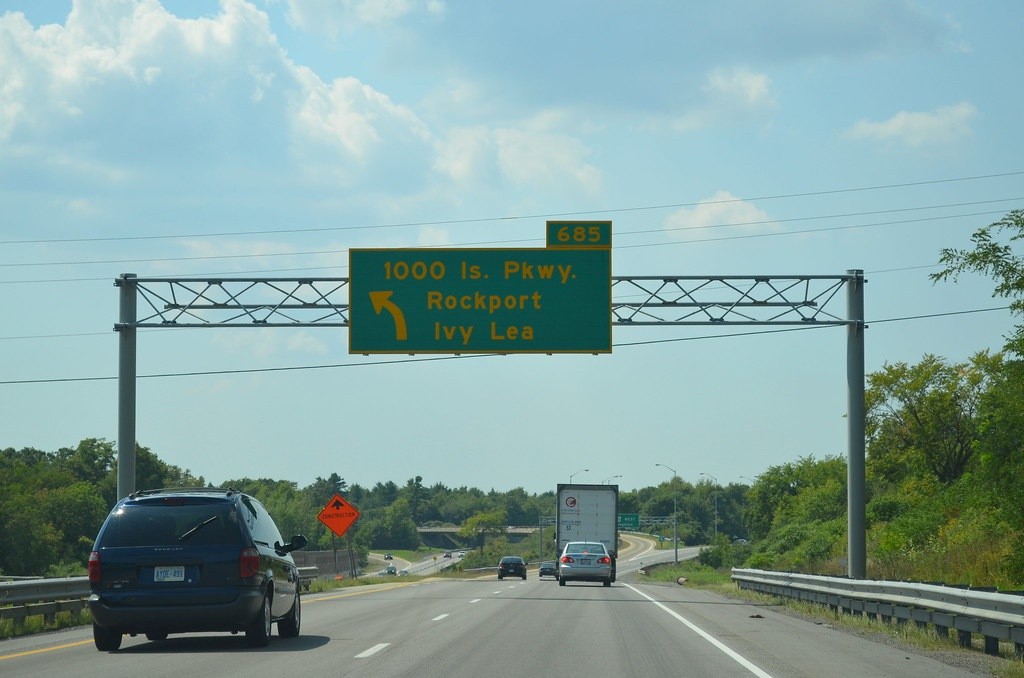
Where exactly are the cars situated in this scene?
[458,551,468,557]
[383,553,393,560]
[398,570,408,577]
[385,566,397,576]
[497,556,528,580]
[557,541,613,587]
[443,551,452,558]
[538,560,559,578]
[507,526,516,530]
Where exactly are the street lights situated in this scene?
[699,472,718,534]
[570,468,589,483]
[601,475,622,485]
[656,463,678,561]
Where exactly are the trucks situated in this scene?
[553,483,620,581]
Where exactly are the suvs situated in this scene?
[87,488,308,652]
